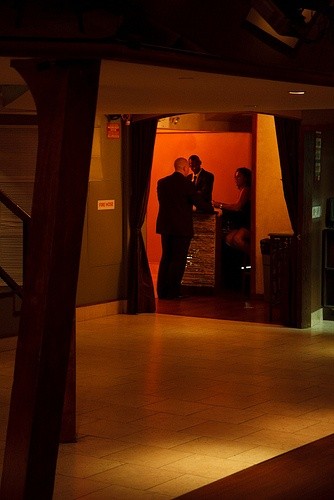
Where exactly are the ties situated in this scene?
[192,176,197,183]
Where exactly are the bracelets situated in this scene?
[219,201,223,209]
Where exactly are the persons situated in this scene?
[187,155,215,212]
[213,167,252,256]
[156,157,223,299]
